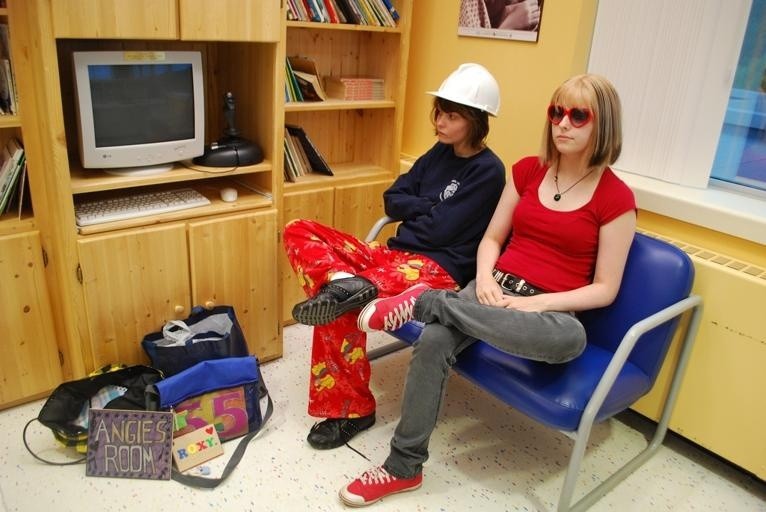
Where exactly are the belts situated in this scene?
[490,268,547,297]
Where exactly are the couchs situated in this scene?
[352,193,703,512]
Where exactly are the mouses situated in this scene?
[220,187,238,202]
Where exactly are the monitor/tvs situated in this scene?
[72,51,205,178]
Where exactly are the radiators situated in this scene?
[625,227,766,481]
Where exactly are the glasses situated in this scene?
[546,105,598,128]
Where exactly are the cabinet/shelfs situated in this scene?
[0,0,65,413]
[282,0,413,332]
[4,0,283,389]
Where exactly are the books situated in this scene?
[285,55,329,102]
[283,124,333,183]
[1,137,31,220]
[324,75,383,101]
[286,1,400,27]
[0,22,18,117]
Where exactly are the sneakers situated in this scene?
[339,465,423,507]
[357,284,433,333]
[308,412,376,449]
[293,275,379,326]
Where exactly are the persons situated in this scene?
[337,74,638,506]
[459,0,542,32]
[281,64,507,451]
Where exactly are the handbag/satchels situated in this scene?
[142,306,249,376]
[22,363,167,465]
[144,356,273,488]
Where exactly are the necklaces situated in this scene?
[553,155,594,202]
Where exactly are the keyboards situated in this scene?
[75,187,211,226]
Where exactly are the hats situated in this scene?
[425,63,500,118]
